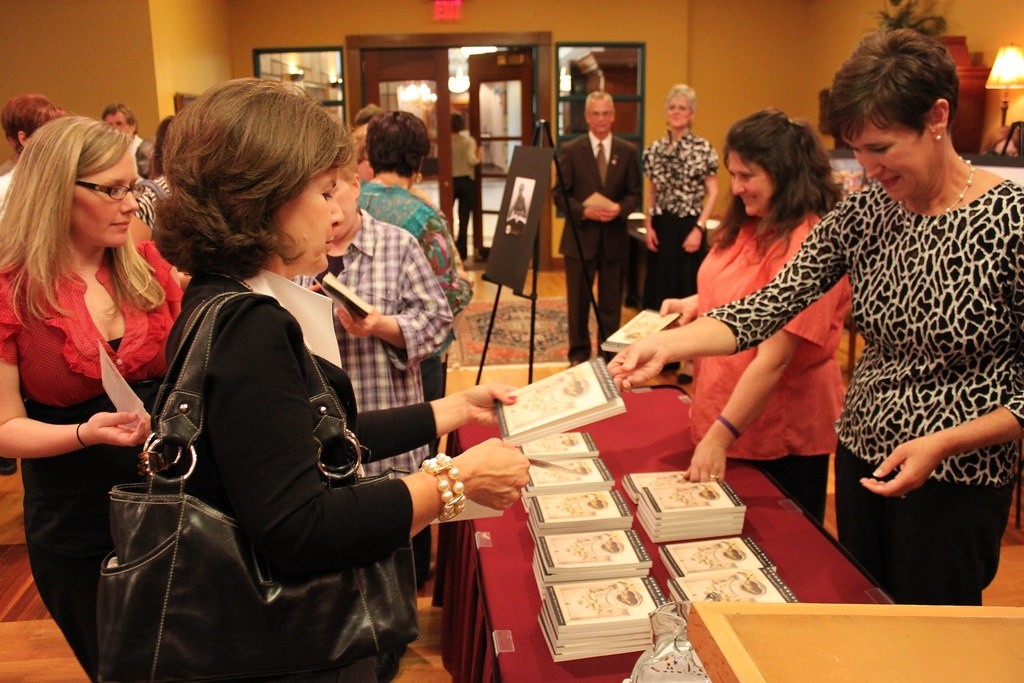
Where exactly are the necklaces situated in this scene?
[899,156,975,231]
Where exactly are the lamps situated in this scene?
[984,43,1024,126]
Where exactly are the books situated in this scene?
[493,356,626,445]
[322,271,374,320]
[520,433,799,662]
[601,308,683,353]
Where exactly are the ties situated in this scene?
[596,142,608,185]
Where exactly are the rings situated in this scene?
[710,475,720,479]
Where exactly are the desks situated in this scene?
[431,387,899,683]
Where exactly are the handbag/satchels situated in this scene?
[97,295,422,682]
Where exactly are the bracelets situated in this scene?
[76,423,87,448]
[696,222,704,231]
[717,415,740,438]
[419,451,466,523]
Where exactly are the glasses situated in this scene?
[75,178,146,201]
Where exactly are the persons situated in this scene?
[659,107,852,526]
[985,126,1020,157]
[0,94,62,474]
[154,78,529,572]
[350,105,475,590]
[433,113,481,260]
[0,117,182,682]
[104,104,191,293]
[642,84,721,384]
[607,31,1024,604]
[559,91,640,365]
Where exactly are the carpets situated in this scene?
[455,296,597,366]
